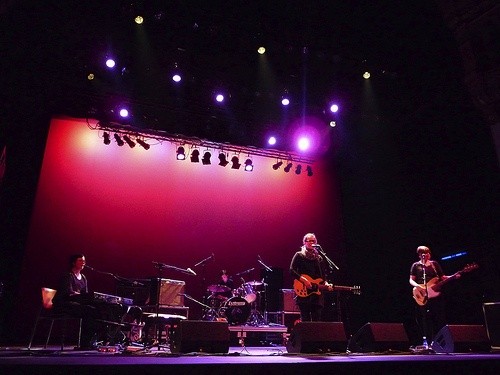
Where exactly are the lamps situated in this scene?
[284,160,292,172]
[306,165,313,176]
[190,145,200,162]
[102,128,111,145]
[202,147,211,165]
[114,132,124,146]
[218,150,229,167]
[176,145,185,160]
[231,152,241,169]
[273,158,283,170]
[244,154,253,171]
[136,136,150,150]
[295,164,301,174]
[123,133,135,148]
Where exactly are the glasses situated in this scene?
[422,250,432,254]
[305,239,315,242]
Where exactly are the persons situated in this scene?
[215,269,234,299]
[55,253,98,351]
[289,233,333,322]
[409,245,462,354]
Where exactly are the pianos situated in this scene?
[63,290,135,351]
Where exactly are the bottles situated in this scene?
[422,337,428,348]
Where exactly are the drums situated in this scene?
[223,296,252,325]
[202,296,225,314]
[236,283,258,304]
[245,281,264,293]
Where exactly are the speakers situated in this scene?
[431,302,500,354]
[349,323,410,353]
[280,288,301,312]
[286,322,347,354]
[152,278,186,306]
[170,320,230,354]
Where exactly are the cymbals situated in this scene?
[206,284,232,292]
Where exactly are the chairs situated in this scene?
[28,287,82,350]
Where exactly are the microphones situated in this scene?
[177,293,186,297]
[84,265,93,270]
[311,244,320,248]
[187,268,197,275]
[422,254,425,259]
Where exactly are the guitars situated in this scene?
[412,263,479,306]
[293,274,362,298]
